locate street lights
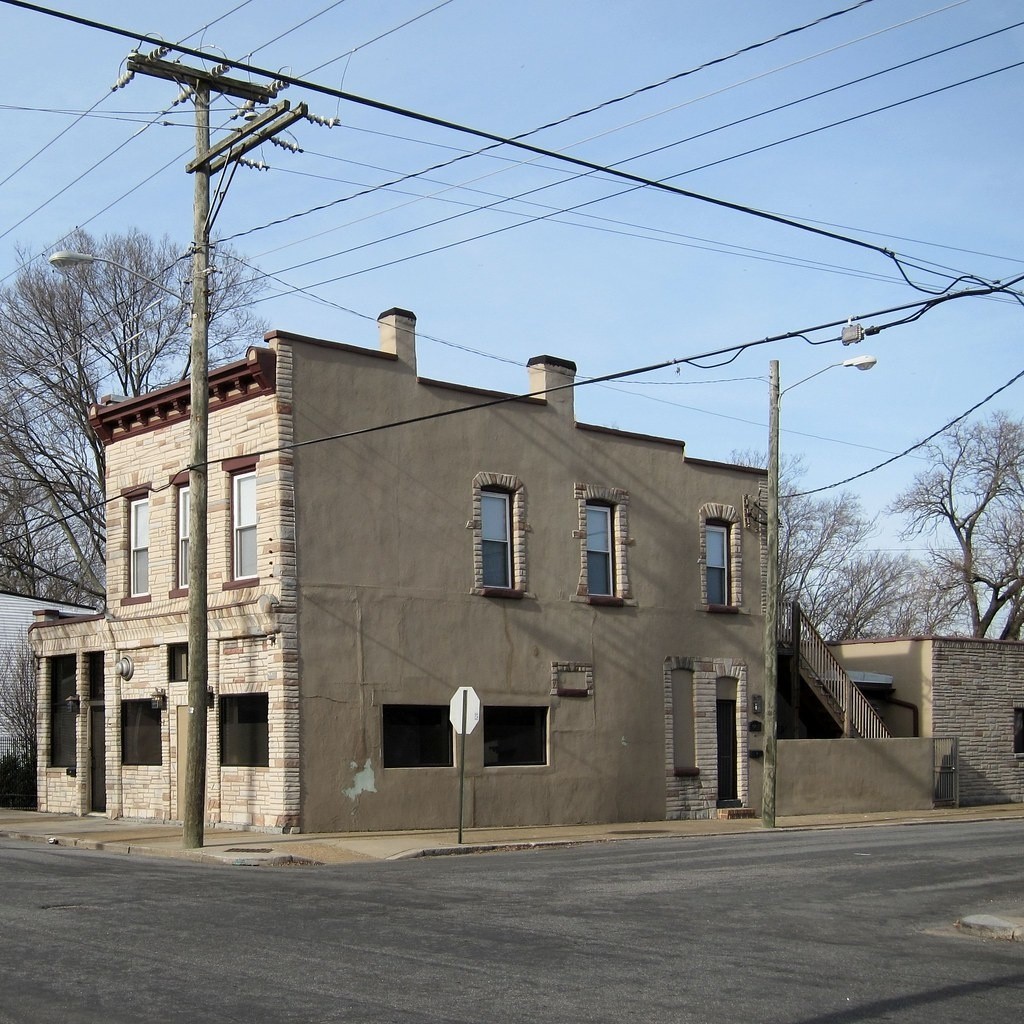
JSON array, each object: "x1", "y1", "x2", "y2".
[
  {"x1": 762, "y1": 355, "x2": 878, "y2": 829},
  {"x1": 45, "y1": 248, "x2": 212, "y2": 852}
]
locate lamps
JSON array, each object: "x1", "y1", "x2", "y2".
[
  {"x1": 66, "y1": 695, "x2": 79, "y2": 713},
  {"x1": 151, "y1": 689, "x2": 165, "y2": 708},
  {"x1": 205, "y1": 686, "x2": 213, "y2": 706}
]
